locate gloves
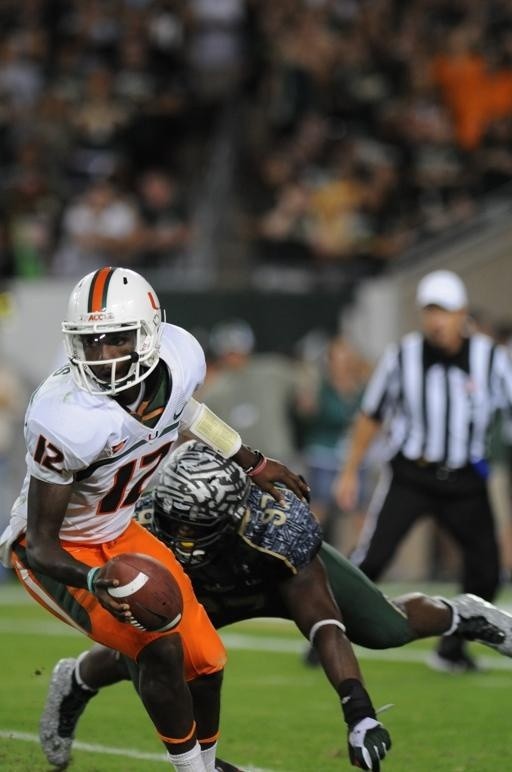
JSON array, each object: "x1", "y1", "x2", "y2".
[{"x1": 341, "y1": 696, "x2": 391, "y2": 771}]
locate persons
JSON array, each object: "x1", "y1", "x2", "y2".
[
  {"x1": 303, "y1": 266, "x2": 511, "y2": 673},
  {"x1": 0, "y1": 2, "x2": 511, "y2": 551},
  {"x1": 1, "y1": 265, "x2": 312, "y2": 772},
  {"x1": 36, "y1": 439, "x2": 512, "y2": 772}
]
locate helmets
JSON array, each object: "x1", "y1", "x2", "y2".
[
  {"x1": 152, "y1": 439, "x2": 252, "y2": 572},
  {"x1": 62, "y1": 266, "x2": 162, "y2": 397}
]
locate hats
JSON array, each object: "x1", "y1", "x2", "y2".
[{"x1": 418, "y1": 273, "x2": 467, "y2": 309}]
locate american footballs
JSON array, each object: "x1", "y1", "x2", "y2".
[{"x1": 106, "y1": 552, "x2": 183, "y2": 633}]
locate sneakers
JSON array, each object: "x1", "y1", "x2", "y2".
[
  {"x1": 444, "y1": 595, "x2": 512, "y2": 658},
  {"x1": 428, "y1": 649, "x2": 488, "y2": 675},
  {"x1": 38, "y1": 650, "x2": 99, "y2": 766}
]
